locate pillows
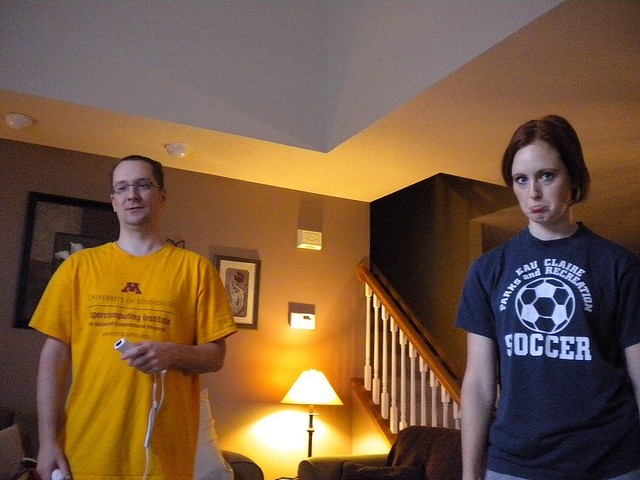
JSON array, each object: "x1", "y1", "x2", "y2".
[
  {"x1": 192, "y1": 389, "x2": 234, "y2": 480},
  {"x1": 342, "y1": 462, "x2": 411, "y2": 480},
  {"x1": 0, "y1": 425, "x2": 25, "y2": 480}
]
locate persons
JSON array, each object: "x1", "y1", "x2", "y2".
[
  {"x1": 455, "y1": 113, "x2": 635, "y2": 478},
  {"x1": 25, "y1": 153, "x2": 238, "y2": 475}
]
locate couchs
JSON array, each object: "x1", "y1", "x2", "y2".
[
  {"x1": 298, "y1": 424, "x2": 465, "y2": 480},
  {"x1": 1, "y1": 410, "x2": 265, "y2": 480}
]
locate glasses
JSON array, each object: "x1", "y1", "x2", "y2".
[{"x1": 110, "y1": 180, "x2": 161, "y2": 193}]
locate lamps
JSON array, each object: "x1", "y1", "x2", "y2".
[
  {"x1": 5, "y1": 113, "x2": 35, "y2": 131},
  {"x1": 281, "y1": 371, "x2": 344, "y2": 457},
  {"x1": 165, "y1": 142, "x2": 190, "y2": 158}
]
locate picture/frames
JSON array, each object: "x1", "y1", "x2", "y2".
[
  {"x1": 14, "y1": 194, "x2": 122, "y2": 330},
  {"x1": 213, "y1": 254, "x2": 260, "y2": 330}
]
